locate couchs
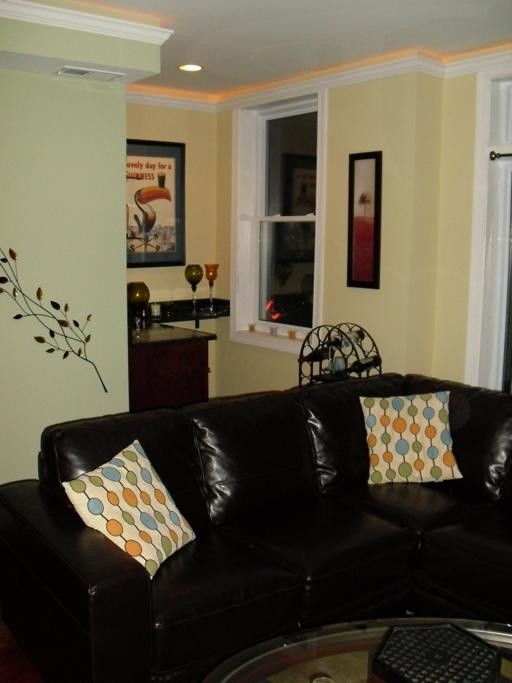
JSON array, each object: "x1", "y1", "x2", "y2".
[{"x1": 1, "y1": 372, "x2": 512, "y2": 682}]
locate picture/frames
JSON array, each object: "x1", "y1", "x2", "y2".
[
  {"x1": 347, "y1": 150, "x2": 381, "y2": 289},
  {"x1": 126, "y1": 138, "x2": 186, "y2": 267}
]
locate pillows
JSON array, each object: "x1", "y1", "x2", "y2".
[
  {"x1": 60, "y1": 438, "x2": 196, "y2": 579},
  {"x1": 359, "y1": 391, "x2": 463, "y2": 486}
]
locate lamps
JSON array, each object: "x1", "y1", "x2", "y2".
[
  {"x1": 204, "y1": 263, "x2": 219, "y2": 312},
  {"x1": 127, "y1": 282, "x2": 150, "y2": 341},
  {"x1": 185, "y1": 263, "x2": 203, "y2": 313}
]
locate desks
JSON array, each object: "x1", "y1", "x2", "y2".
[{"x1": 128, "y1": 322, "x2": 216, "y2": 413}]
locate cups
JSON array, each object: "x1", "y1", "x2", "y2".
[{"x1": 151, "y1": 301, "x2": 161, "y2": 317}]
